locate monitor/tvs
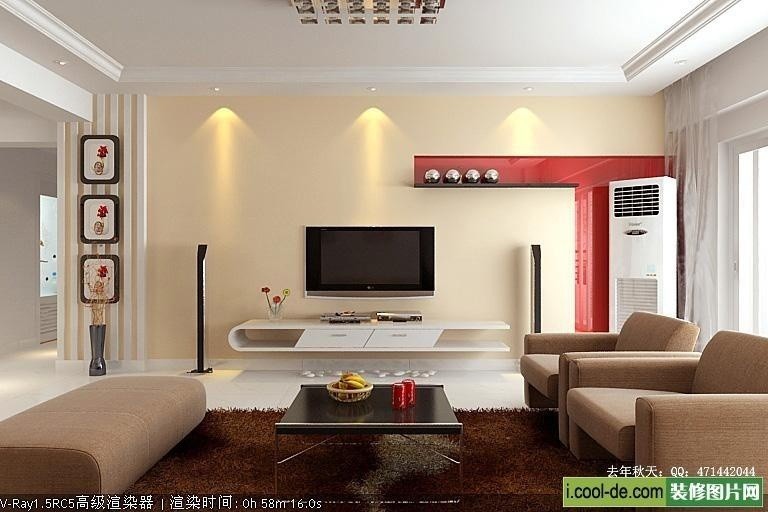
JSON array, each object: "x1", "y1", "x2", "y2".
[{"x1": 305, "y1": 226, "x2": 435, "y2": 300}]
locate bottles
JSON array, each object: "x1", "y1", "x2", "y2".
[{"x1": 392, "y1": 379, "x2": 416, "y2": 408}]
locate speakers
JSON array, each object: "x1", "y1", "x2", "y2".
[
  {"x1": 189, "y1": 245, "x2": 212, "y2": 373},
  {"x1": 530, "y1": 245, "x2": 541, "y2": 334}
]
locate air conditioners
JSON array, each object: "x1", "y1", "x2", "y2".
[{"x1": 609, "y1": 176, "x2": 678, "y2": 333}]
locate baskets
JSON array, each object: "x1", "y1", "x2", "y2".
[{"x1": 327, "y1": 379, "x2": 373, "y2": 402}]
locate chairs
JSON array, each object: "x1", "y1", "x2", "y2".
[
  {"x1": 566, "y1": 329, "x2": 768, "y2": 495},
  {"x1": 521, "y1": 311, "x2": 701, "y2": 448}
]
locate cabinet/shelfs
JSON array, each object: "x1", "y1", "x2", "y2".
[{"x1": 227, "y1": 319, "x2": 511, "y2": 353}]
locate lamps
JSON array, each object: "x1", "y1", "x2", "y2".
[{"x1": 290, "y1": 0, "x2": 446, "y2": 26}]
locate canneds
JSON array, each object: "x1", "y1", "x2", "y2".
[
  {"x1": 392, "y1": 383, "x2": 405, "y2": 410},
  {"x1": 402, "y1": 380, "x2": 416, "y2": 407}
]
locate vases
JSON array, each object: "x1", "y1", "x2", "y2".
[{"x1": 88, "y1": 324, "x2": 107, "y2": 376}]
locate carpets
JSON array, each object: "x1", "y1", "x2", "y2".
[{"x1": 112, "y1": 406, "x2": 636, "y2": 512}]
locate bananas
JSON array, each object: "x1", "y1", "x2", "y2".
[{"x1": 340, "y1": 373, "x2": 366, "y2": 389}]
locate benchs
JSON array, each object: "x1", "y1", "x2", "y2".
[{"x1": 0, "y1": 375, "x2": 207, "y2": 494}]
